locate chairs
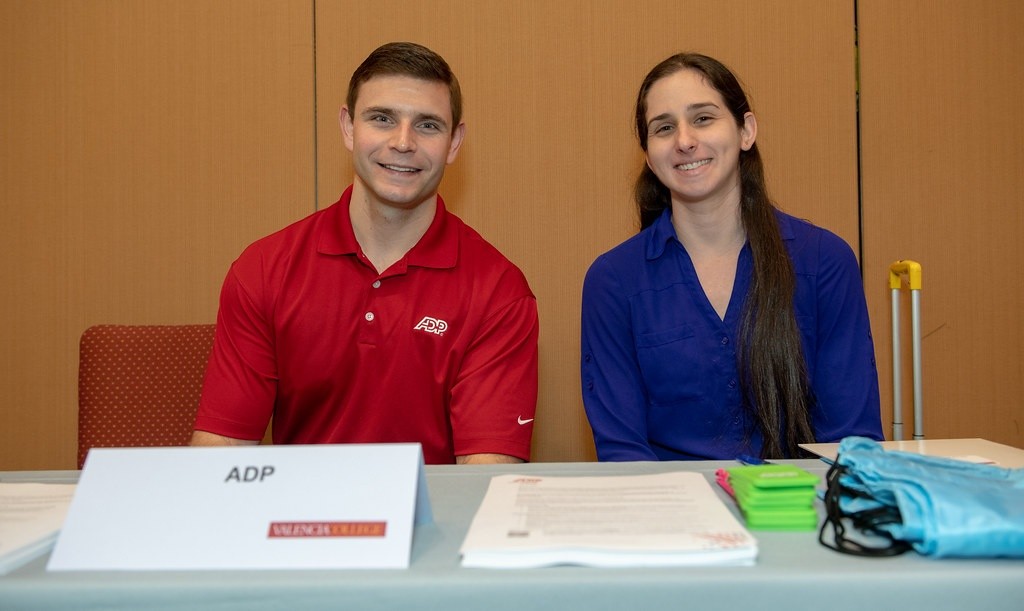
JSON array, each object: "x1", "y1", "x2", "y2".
[{"x1": 77, "y1": 324, "x2": 217, "y2": 470}]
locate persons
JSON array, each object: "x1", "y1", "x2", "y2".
[
  {"x1": 580, "y1": 52, "x2": 885, "y2": 462},
  {"x1": 193, "y1": 43, "x2": 540, "y2": 466}
]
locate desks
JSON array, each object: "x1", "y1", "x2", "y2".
[{"x1": 0, "y1": 437, "x2": 1024, "y2": 611}]
